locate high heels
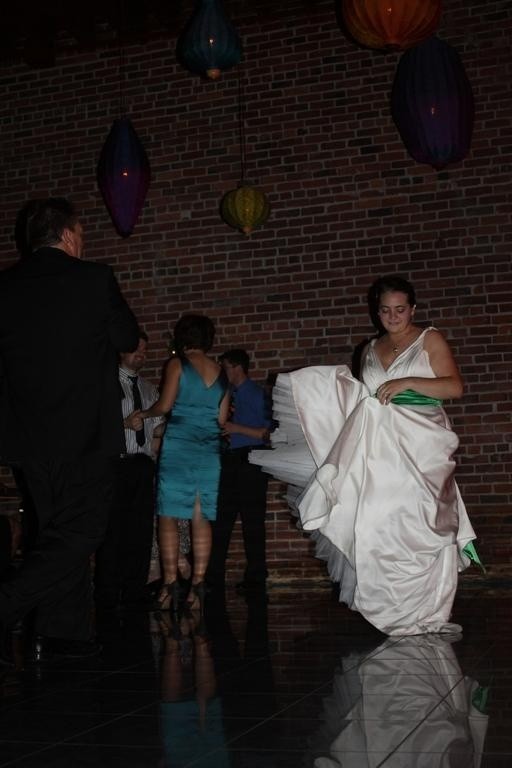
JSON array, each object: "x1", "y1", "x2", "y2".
[
  {"x1": 183, "y1": 579, "x2": 206, "y2": 614},
  {"x1": 152, "y1": 579, "x2": 181, "y2": 615}
]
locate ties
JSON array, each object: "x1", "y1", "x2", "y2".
[{"x1": 128, "y1": 375, "x2": 146, "y2": 447}]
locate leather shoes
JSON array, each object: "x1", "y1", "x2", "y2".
[{"x1": 235, "y1": 579, "x2": 265, "y2": 591}]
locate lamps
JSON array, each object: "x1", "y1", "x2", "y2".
[
  {"x1": 219, "y1": 181, "x2": 272, "y2": 237},
  {"x1": 340, "y1": 2, "x2": 444, "y2": 51},
  {"x1": 389, "y1": 33, "x2": 476, "y2": 169},
  {"x1": 173, "y1": 3, "x2": 242, "y2": 81},
  {"x1": 95, "y1": 116, "x2": 153, "y2": 241}
]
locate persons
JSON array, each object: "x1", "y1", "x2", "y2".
[
  {"x1": 250, "y1": 273, "x2": 489, "y2": 638},
  {"x1": 4, "y1": 197, "x2": 142, "y2": 678},
  {"x1": 111, "y1": 316, "x2": 277, "y2": 619},
  {"x1": 156, "y1": 609, "x2": 231, "y2": 767},
  {"x1": 307, "y1": 636, "x2": 494, "y2": 767},
  {"x1": 383, "y1": 334, "x2": 406, "y2": 354}
]
